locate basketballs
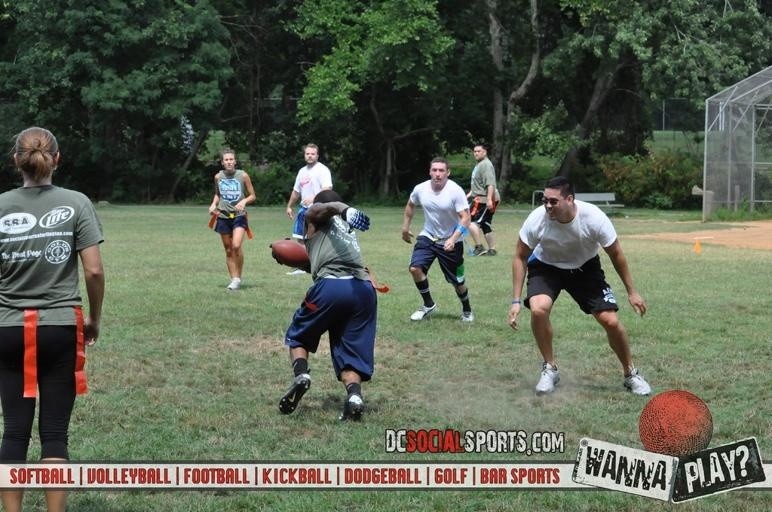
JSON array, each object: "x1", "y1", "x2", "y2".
[{"x1": 639, "y1": 389, "x2": 713, "y2": 457}]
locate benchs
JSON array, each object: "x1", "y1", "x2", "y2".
[{"x1": 533, "y1": 190, "x2": 625, "y2": 214}]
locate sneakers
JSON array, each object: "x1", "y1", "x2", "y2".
[
  {"x1": 473, "y1": 244, "x2": 487, "y2": 256},
  {"x1": 227, "y1": 279, "x2": 240, "y2": 289},
  {"x1": 279, "y1": 373, "x2": 311, "y2": 414},
  {"x1": 536, "y1": 362, "x2": 560, "y2": 394},
  {"x1": 488, "y1": 249, "x2": 496, "y2": 256},
  {"x1": 410, "y1": 302, "x2": 437, "y2": 321},
  {"x1": 286, "y1": 268, "x2": 306, "y2": 274},
  {"x1": 461, "y1": 312, "x2": 473, "y2": 321},
  {"x1": 624, "y1": 368, "x2": 651, "y2": 395},
  {"x1": 339, "y1": 392, "x2": 364, "y2": 421}
]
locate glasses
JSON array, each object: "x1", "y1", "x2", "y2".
[{"x1": 541, "y1": 196, "x2": 559, "y2": 205}]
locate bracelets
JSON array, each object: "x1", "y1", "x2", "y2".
[{"x1": 511, "y1": 298, "x2": 522, "y2": 304}]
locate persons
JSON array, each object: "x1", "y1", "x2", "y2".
[
  {"x1": 0, "y1": 126, "x2": 106, "y2": 512},
  {"x1": 209, "y1": 149, "x2": 256, "y2": 290},
  {"x1": 509, "y1": 178, "x2": 650, "y2": 397},
  {"x1": 402, "y1": 157, "x2": 473, "y2": 323},
  {"x1": 466, "y1": 145, "x2": 500, "y2": 256},
  {"x1": 286, "y1": 143, "x2": 333, "y2": 275},
  {"x1": 279, "y1": 189, "x2": 378, "y2": 422}
]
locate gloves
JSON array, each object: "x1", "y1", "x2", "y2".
[{"x1": 341, "y1": 207, "x2": 370, "y2": 233}]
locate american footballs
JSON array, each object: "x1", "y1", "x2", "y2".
[{"x1": 271, "y1": 240, "x2": 309, "y2": 267}]
